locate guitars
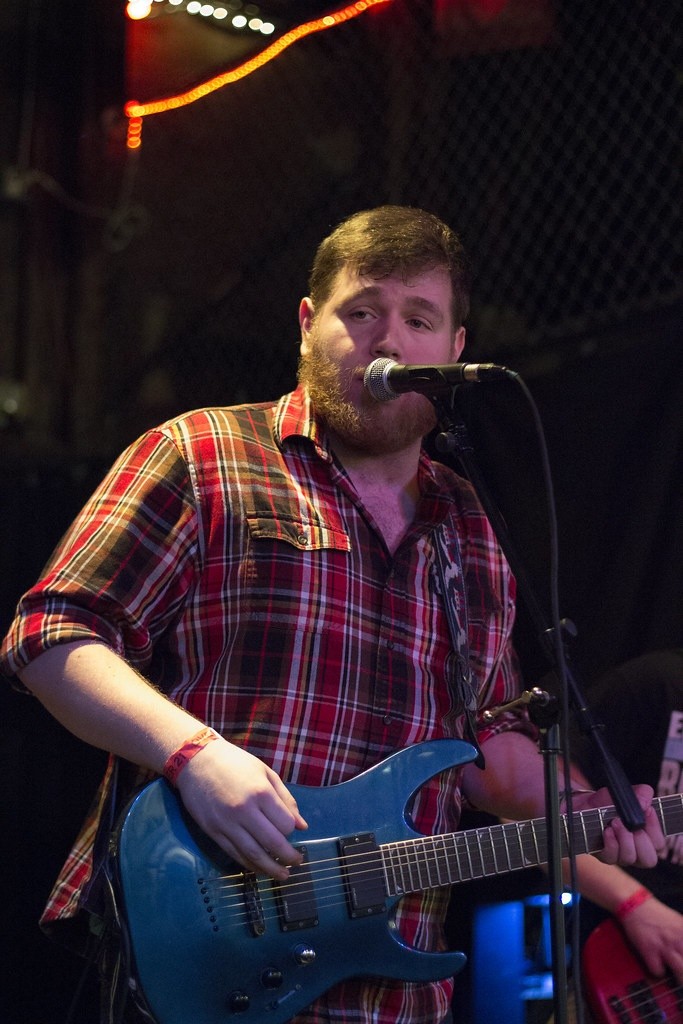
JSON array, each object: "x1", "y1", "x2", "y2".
[
  {"x1": 579, "y1": 899, "x2": 683, "y2": 1024},
  {"x1": 106, "y1": 737, "x2": 683, "y2": 1024}
]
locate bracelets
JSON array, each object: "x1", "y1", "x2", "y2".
[
  {"x1": 613, "y1": 886, "x2": 653, "y2": 919},
  {"x1": 163, "y1": 728, "x2": 216, "y2": 784}
]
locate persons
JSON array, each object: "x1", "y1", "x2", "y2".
[{"x1": 1, "y1": 202, "x2": 682, "y2": 1023}]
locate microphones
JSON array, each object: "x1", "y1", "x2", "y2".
[{"x1": 363, "y1": 357, "x2": 508, "y2": 402}]
